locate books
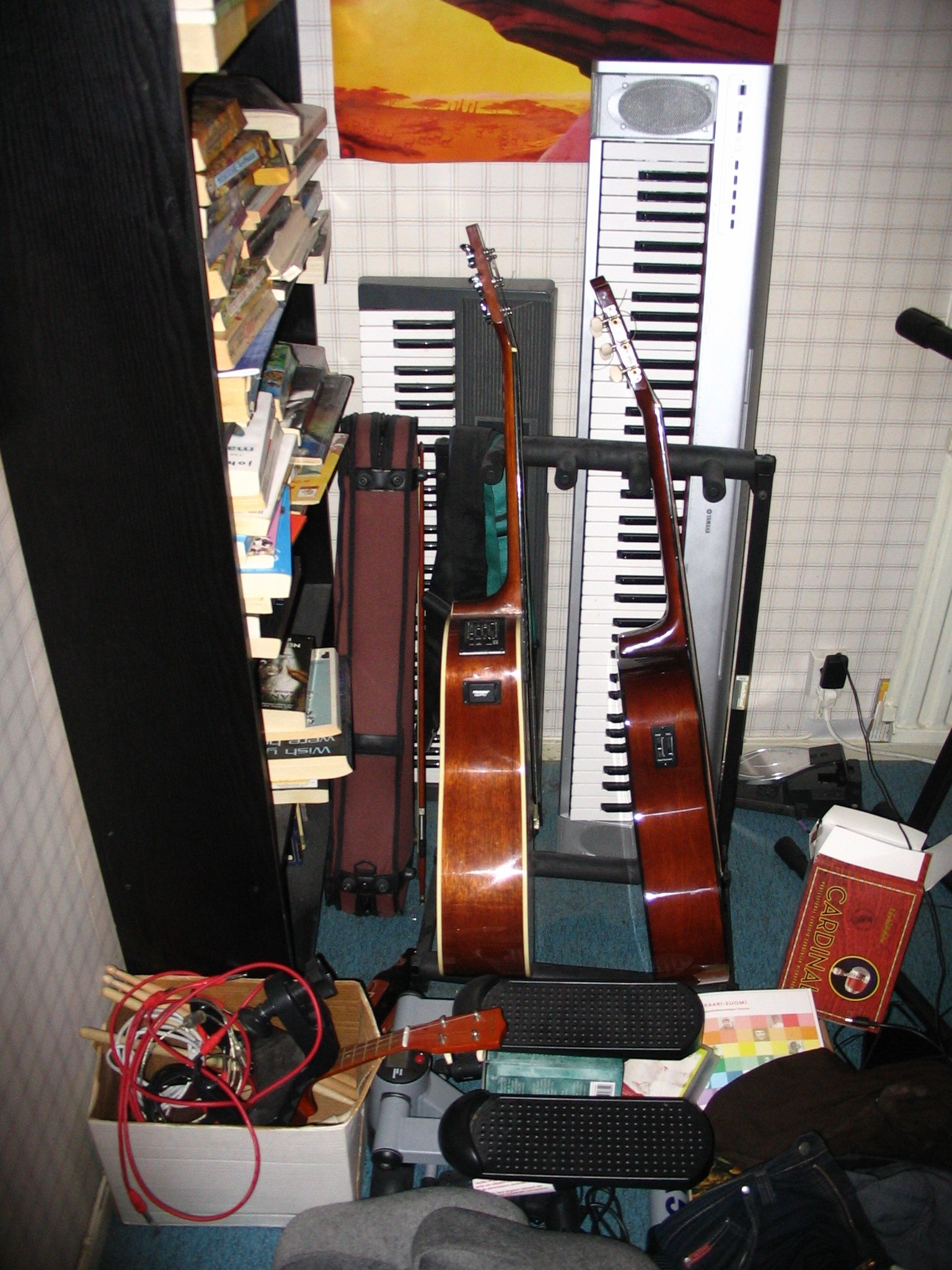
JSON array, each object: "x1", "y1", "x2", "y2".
[{"x1": 175, "y1": 0, "x2": 356, "y2": 806}]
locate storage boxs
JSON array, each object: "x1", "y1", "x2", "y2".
[
  {"x1": 85, "y1": 975, "x2": 385, "y2": 1228},
  {"x1": 779, "y1": 806, "x2": 951, "y2": 1034}
]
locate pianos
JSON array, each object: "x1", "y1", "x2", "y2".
[
  {"x1": 357, "y1": 276, "x2": 554, "y2": 805},
  {"x1": 560, "y1": 58, "x2": 773, "y2": 826}
]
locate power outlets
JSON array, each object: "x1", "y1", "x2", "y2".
[{"x1": 807, "y1": 650, "x2": 848, "y2": 699}]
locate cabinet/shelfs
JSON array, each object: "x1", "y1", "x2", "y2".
[{"x1": 1, "y1": 0, "x2": 332, "y2": 975}]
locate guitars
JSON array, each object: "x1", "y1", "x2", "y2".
[
  {"x1": 292, "y1": 1006, "x2": 507, "y2": 1124},
  {"x1": 434, "y1": 224, "x2": 539, "y2": 980},
  {"x1": 588, "y1": 276, "x2": 733, "y2": 985}
]
